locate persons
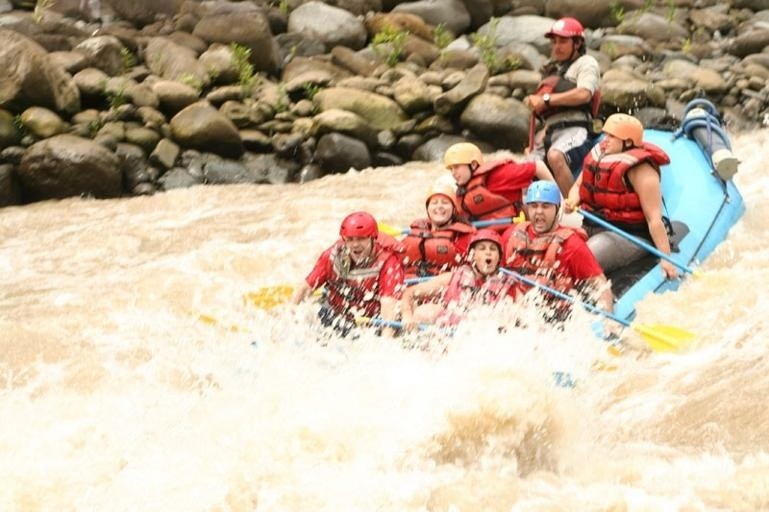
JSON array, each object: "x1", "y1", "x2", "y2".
[
  {"x1": 400, "y1": 185, "x2": 477, "y2": 307},
  {"x1": 503, "y1": 179, "x2": 622, "y2": 337},
  {"x1": 397, "y1": 230, "x2": 524, "y2": 351},
  {"x1": 523, "y1": 16, "x2": 601, "y2": 198},
  {"x1": 291, "y1": 211, "x2": 403, "y2": 339},
  {"x1": 443, "y1": 142, "x2": 564, "y2": 236},
  {"x1": 561, "y1": 114, "x2": 678, "y2": 282}
]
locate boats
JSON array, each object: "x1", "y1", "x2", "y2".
[{"x1": 529, "y1": 121, "x2": 754, "y2": 388}]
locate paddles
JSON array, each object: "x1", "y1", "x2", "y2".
[
  {"x1": 250, "y1": 282, "x2": 334, "y2": 313},
  {"x1": 575, "y1": 208, "x2": 725, "y2": 291},
  {"x1": 375, "y1": 208, "x2": 528, "y2": 242},
  {"x1": 492, "y1": 264, "x2": 693, "y2": 355}
]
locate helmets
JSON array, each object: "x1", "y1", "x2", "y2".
[
  {"x1": 443, "y1": 142, "x2": 483, "y2": 169},
  {"x1": 339, "y1": 212, "x2": 378, "y2": 239},
  {"x1": 544, "y1": 17, "x2": 585, "y2": 38},
  {"x1": 603, "y1": 113, "x2": 643, "y2": 147},
  {"x1": 426, "y1": 183, "x2": 458, "y2": 216},
  {"x1": 526, "y1": 180, "x2": 561, "y2": 210},
  {"x1": 467, "y1": 228, "x2": 502, "y2": 260}
]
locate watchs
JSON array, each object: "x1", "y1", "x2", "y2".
[{"x1": 543, "y1": 92, "x2": 551, "y2": 109}]
